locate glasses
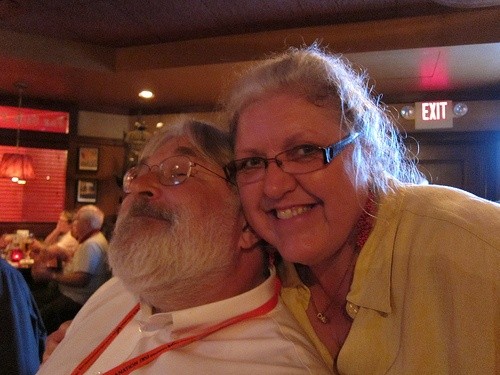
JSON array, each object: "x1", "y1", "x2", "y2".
[
  {"x1": 221, "y1": 132, "x2": 363, "y2": 185},
  {"x1": 119, "y1": 156, "x2": 237, "y2": 193}
]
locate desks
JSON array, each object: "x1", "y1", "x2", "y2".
[{"x1": 0, "y1": 236, "x2": 58, "y2": 301}]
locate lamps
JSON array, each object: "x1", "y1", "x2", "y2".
[
  {"x1": 125, "y1": 100, "x2": 153, "y2": 154},
  {"x1": 0, "y1": 82, "x2": 38, "y2": 181},
  {"x1": 400, "y1": 99, "x2": 468, "y2": 130}
]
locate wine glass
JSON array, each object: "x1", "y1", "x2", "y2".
[{"x1": 24, "y1": 237, "x2": 32, "y2": 259}]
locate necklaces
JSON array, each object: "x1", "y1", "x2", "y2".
[{"x1": 308, "y1": 243, "x2": 358, "y2": 324}]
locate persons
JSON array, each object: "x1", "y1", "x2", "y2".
[
  {"x1": 223, "y1": 44, "x2": 500, "y2": 375},
  {"x1": 36, "y1": 118, "x2": 332, "y2": 375},
  {"x1": 0, "y1": 205, "x2": 113, "y2": 375}
]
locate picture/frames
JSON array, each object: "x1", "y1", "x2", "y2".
[
  {"x1": 74, "y1": 177, "x2": 99, "y2": 205},
  {"x1": 78, "y1": 145, "x2": 101, "y2": 174}
]
